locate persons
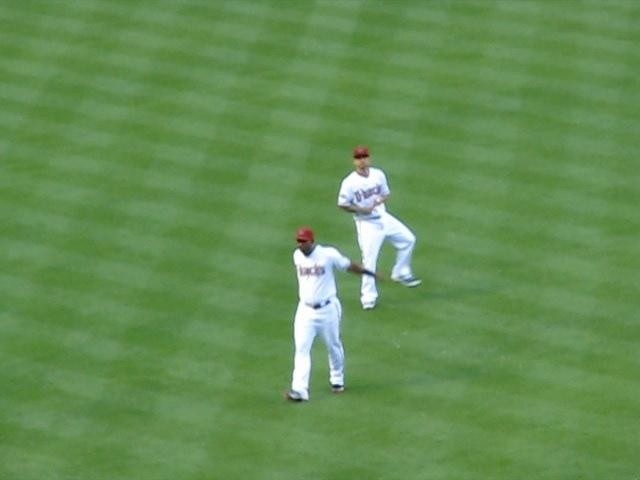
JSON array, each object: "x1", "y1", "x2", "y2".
[
  {"x1": 284, "y1": 228, "x2": 383, "y2": 401},
  {"x1": 337, "y1": 146, "x2": 424, "y2": 310}
]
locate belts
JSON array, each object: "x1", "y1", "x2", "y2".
[{"x1": 307, "y1": 301, "x2": 330, "y2": 309}]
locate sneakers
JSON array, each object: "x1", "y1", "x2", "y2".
[
  {"x1": 362, "y1": 302, "x2": 375, "y2": 309},
  {"x1": 285, "y1": 390, "x2": 301, "y2": 400},
  {"x1": 332, "y1": 384, "x2": 344, "y2": 392},
  {"x1": 391, "y1": 275, "x2": 422, "y2": 286}
]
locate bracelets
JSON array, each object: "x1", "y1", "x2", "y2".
[
  {"x1": 362, "y1": 270, "x2": 376, "y2": 278},
  {"x1": 375, "y1": 198, "x2": 381, "y2": 206}
]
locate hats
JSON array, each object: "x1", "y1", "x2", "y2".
[
  {"x1": 297, "y1": 229, "x2": 313, "y2": 241},
  {"x1": 353, "y1": 148, "x2": 369, "y2": 157}
]
